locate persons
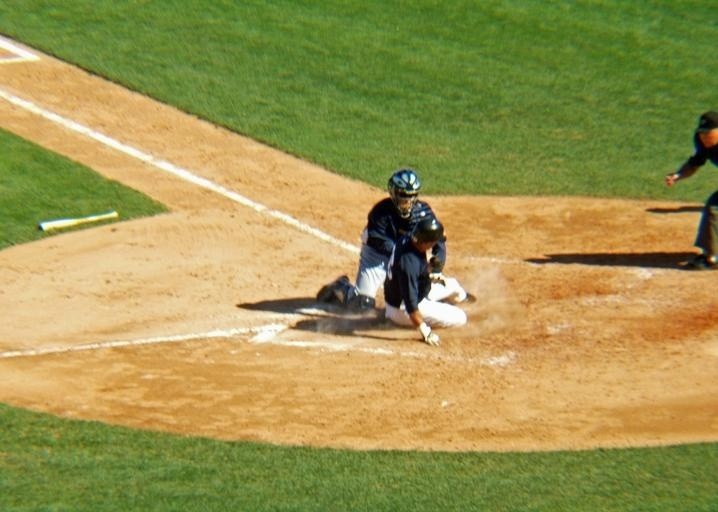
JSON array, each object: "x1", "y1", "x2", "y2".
[
  {"x1": 383, "y1": 216, "x2": 468, "y2": 346},
  {"x1": 664, "y1": 111, "x2": 718, "y2": 271},
  {"x1": 316, "y1": 168, "x2": 446, "y2": 315}
]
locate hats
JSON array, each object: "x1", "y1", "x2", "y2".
[{"x1": 695, "y1": 111, "x2": 718, "y2": 134}]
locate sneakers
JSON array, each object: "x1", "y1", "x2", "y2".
[
  {"x1": 316, "y1": 274, "x2": 350, "y2": 304},
  {"x1": 677, "y1": 253, "x2": 717, "y2": 269}
]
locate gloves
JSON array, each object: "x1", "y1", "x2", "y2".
[{"x1": 419, "y1": 323, "x2": 441, "y2": 347}]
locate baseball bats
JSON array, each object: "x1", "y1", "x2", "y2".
[{"x1": 39, "y1": 210, "x2": 119, "y2": 232}]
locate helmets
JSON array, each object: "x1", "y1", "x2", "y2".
[
  {"x1": 414, "y1": 218, "x2": 446, "y2": 244},
  {"x1": 387, "y1": 167, "x2": 421, "y2": 206}
]
